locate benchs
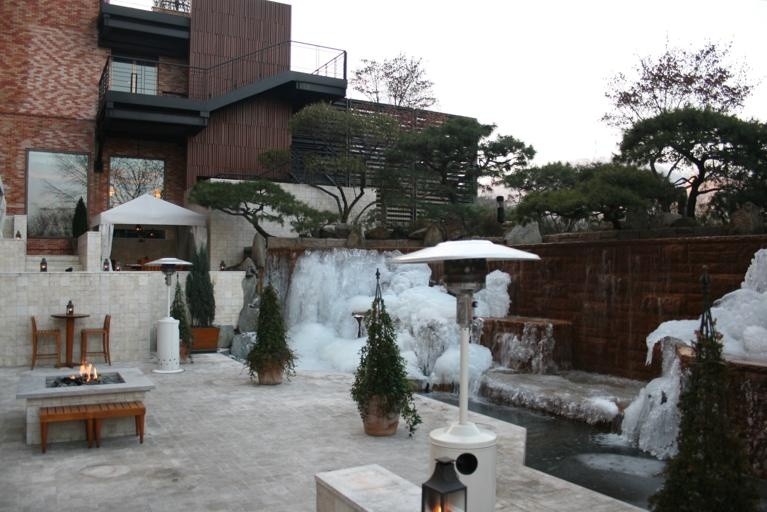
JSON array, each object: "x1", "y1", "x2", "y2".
[{"x1": 39, "y1": 400, "x2": 147, "y2": 454}]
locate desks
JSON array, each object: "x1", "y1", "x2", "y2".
[{"x1": 52, "y1": 313, "x2": 90, "y2": 369}]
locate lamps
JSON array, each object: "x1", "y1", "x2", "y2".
[
  {"x1": 420, "y1": 449, "x2": 479, "y2": 512},
  {"x1": 66, "y1": 300, "x2": 74, "y2": 315},
  {"x1": 40, "y1": 258, "x2": 48, "y2": 272}
]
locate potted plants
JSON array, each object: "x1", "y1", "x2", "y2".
[
  {"x1": 172, "y1": 244, "x2": 223, "y2": 366},
  {"x1": 353, "y1": 297, "x2": 423, "y2": 437},
  {"x1": 246, "y1": 281, "x2": 299, "y2": 385}
]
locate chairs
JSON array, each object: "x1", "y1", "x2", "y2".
[
  {"x1": 30, "y1": 316, "x2": 61, "y2": 370},
  {"x1": 81, "y1": 315, "x2": 113, "y2": 367}
]
